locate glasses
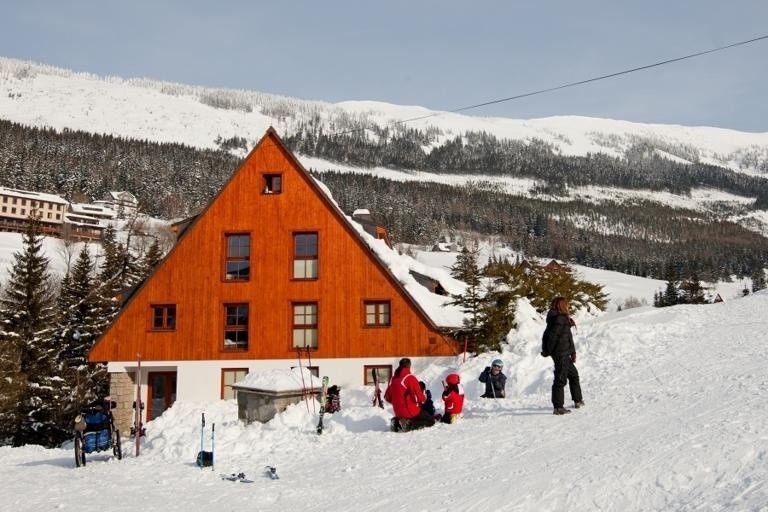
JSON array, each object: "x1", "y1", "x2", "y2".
[{"x1": 493, "y1": 364, "x2": 501, "y2": 368}]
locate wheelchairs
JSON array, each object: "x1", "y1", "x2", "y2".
[{"x1": 72, "y1": 394, "x2": 122, "y2": 468}]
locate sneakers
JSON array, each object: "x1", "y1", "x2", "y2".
[
  {"x1": 391, "y1": 418, "x2": 397, "y2": 432},
  {"x1": 575, "y1": 401, "x2": 584, "y2": 408},
  {"x1": 553, "y1": 407, "x2": 570, "y2": 414},
  {"x1": 398, "y1": 417, "x2": 409, "y2": 432}
]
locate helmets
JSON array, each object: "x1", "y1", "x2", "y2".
[{"x1": 492, "y1": 360, "x2": 504, "y2": 367}]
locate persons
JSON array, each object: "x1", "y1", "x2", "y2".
[
  {"x1": 383, "y1": 358, "x2": 435, "y2": 432},
  {"x1": 479, "y1": 359, "x2": 507, "y2": 398},
  {"x1": 546, "y1": 296, "x2": 586, "y2": 414},
  {"x1": 418, "y1": 380, "x2": 433, "y2": 417},
  {"x1": 441, "y1": 374, "x2": 465, "y2": 424}
]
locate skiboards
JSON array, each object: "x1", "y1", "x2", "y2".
[
  {"x1": 221, "y1": 464, "x2": 280, "y2": 484},
  {"x1": 316, "y1": 377, "x2": 329, "y2": 434},
  {"x1": 371, "y1": 367, "x2": 385, "y2": 408}
]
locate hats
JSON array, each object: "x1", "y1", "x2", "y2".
[{"x1": 446, "y1": 374, "x2": 460, "y2": 385}]
